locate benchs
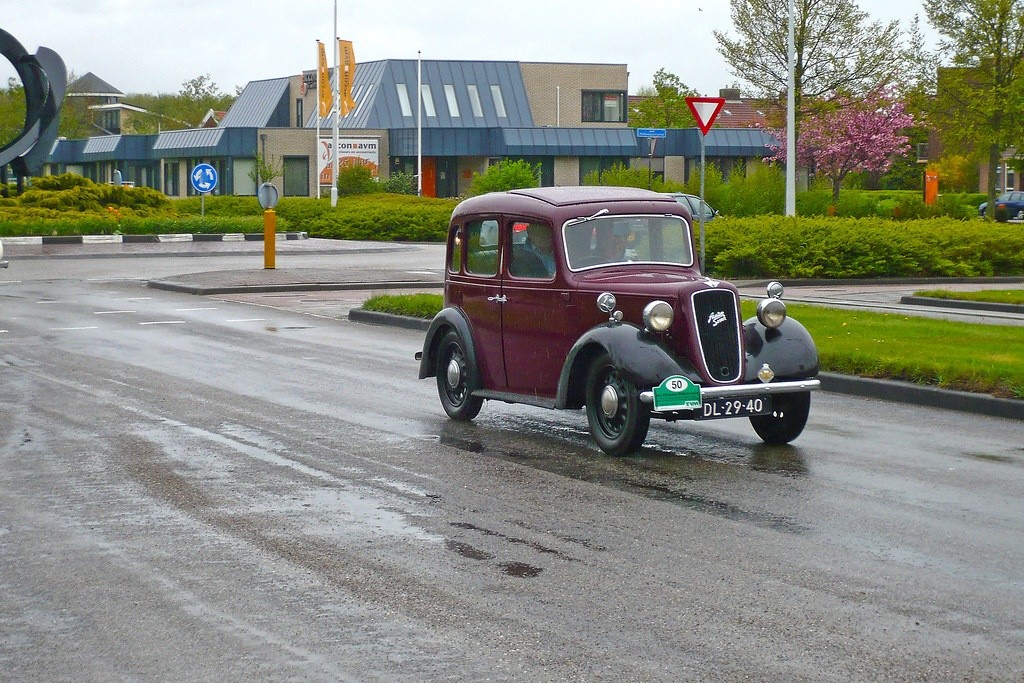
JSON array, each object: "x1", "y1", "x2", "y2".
[{"x1": 469, "y1": 250, "x2": 548, "y2": 279}]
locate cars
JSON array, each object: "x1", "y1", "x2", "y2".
[
  {"x1": 413, "y1": 186, "x2": 821, "y2": 457},
  {"x1": 482, "y1": 219, "x2": 528, "y2": 245},
  {"x1": 978, "y1": 190, "x2": 1024, "y2": 220}
]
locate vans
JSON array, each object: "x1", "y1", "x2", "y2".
[{"x1": 629, "y1": 192, "x2": 721, "y2": 233}]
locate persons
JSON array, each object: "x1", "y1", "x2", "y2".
[{"x1": 518, "y1": 222, "x2": 556, "y2": 277}]
